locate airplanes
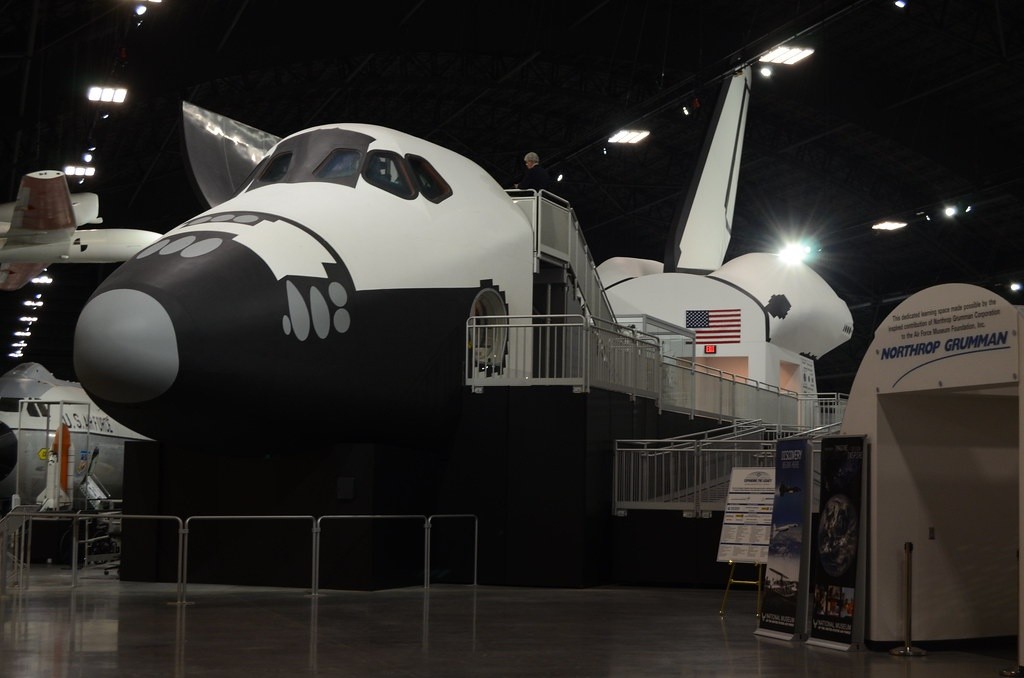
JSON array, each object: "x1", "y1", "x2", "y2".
[
  {"x1": 1, "y1": 44, "x2": 859, "y2": 590},
  {"x1": 0, "y1": 362, "x2": 157, "y2": 571}
]
